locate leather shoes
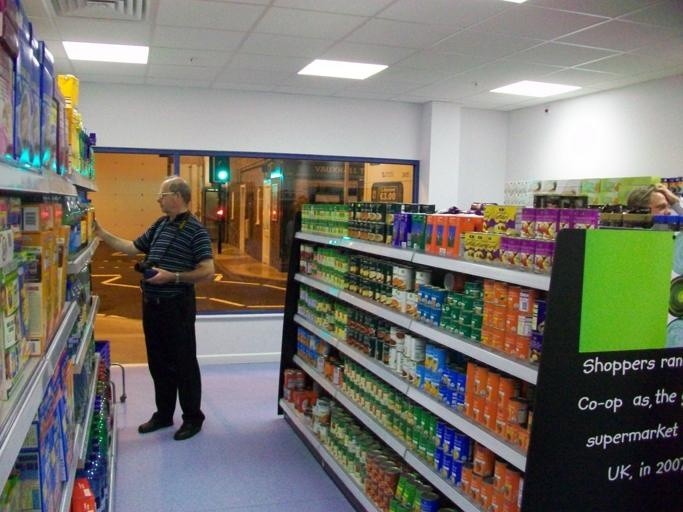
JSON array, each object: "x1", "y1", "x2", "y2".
[
  {"x1": 172, "y1": 424, "x2": 199, "y2": 440},
  {"x1": 137, "y1": 417, "x2": 171, "y2": 434}
]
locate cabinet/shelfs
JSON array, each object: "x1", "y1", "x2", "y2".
[
  {"x1": 0, "y1": 133, "x2": 114, "y2": 512},
  {"x1": 277, "y1": 209, "x2": 683, "y2": 511}
]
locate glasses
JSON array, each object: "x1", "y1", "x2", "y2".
[{"x1": 155, "y1": 189, "x2": 176, "y2": 203}]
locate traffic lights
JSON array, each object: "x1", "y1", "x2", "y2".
[{"x1": 209, "y1": 156, "x2": 231, "y2": 184}]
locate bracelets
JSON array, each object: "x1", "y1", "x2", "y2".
[{"x1": 175, "y1": 272, "x2": 180, "y2": 285}]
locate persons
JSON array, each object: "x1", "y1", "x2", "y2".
[
  {"x1": 626, "y1": 183, "x2": 682, "y2": 217},
  {"x1": 93, "y1": 176, "x2": 216, "y2": 440}
]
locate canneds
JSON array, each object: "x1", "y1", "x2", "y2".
[{"x1": 284, "y1": 196, "x2": 600, "y2": 512}]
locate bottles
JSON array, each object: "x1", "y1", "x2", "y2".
[{"x1": 75, "y1": 355, "x2": 113, "y2": 511}]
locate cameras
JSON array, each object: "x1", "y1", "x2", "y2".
[{"x1": 135, "y1": 260, "x2": 158, "y2": 274}]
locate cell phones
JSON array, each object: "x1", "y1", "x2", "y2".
[{"x1": 144, "y1": 269, "x2": 157, "y2": 279}]
